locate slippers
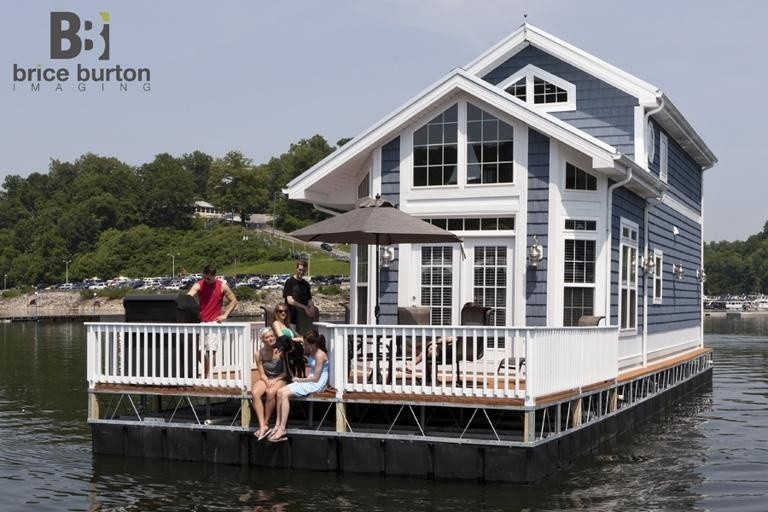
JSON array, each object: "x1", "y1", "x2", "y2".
[{"x1": 254, "y1": 427, "x2": 288, "y2": 443}]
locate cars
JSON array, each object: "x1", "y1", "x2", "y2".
[
  {"x1": 320, "y1": 243, "x2": 332, "y2": 251},
  {"x1": 703, "y1": 292, "x2": 768, "y2": 311},
  {"x1": 35, "y1": 272, "x2": 350, "y2": 292}
]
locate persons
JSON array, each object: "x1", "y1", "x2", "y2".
[
  {"x1": 282, "y1": 260, "x2": 316, "y2": 336},
  {"x1": 249, "y1": 326, "x2": 289, "y2": 442},
  {"x1": 187, "y1": 264, "x2": 239, "y2": 378},
  {"x1": 394, "y1": 334, "x2": 458, "y2": 384},
  {"x1": 271, "y1": 301, "x2": 306, "y2": 347},
  {"x1": 267, "y1": 329, "x2": 330, "y2": 442}
]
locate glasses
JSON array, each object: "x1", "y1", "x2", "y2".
[
  {"x1": 277, "y1": 310, "x2": 288, "y2": 313},
  {"x1": 203, "y1": 276, "x2": 214, "y2": 281}
]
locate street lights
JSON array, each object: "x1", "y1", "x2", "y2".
[
  {"x1": 301, "y1": 251, "x2": 318, "y2": 276},
  {"x1": 63, "y1": 260, "x2": 72, "y2": 284},
  {"x1": 4, "y1": 274, "x2": 8, "y2": 289},
  {"x1": 167, "y1": 252, "x2": 180, "y2": 278}
]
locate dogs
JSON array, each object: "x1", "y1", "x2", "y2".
[{"x1": 272, "y1": 336, "x2": 307, "y2": 378}]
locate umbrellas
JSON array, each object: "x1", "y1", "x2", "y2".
[{"x1": 286, "y1": 194, "x2": 464, "y2": 326}]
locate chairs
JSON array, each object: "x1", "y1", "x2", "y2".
[
  {"x1": 578, "y1": 316, "x2": 605, "y2": 328},
  {"x1": 497, "y1": 357, "x2": 526, "y2": 385},
  {"x1": 258, "y1": 302, "x2": 495, "y2": 386}
]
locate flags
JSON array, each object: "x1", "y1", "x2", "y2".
[{"x1": 222, "y1": 175, "x2": 233, "y2": 185}]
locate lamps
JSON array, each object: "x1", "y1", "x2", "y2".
[
  {"x1": 381, "y1": 245, "x2": 395, "y2": 267},
  {"x1": 673, "y1": 262, "x2": 685, "y2": 279},
  {"x1": 695, "y1": 268, "x2": 707, "y2": 282},
  {"x1": 645, "y1": 255, "x2": 655, "y2": 274},
  {"x1": 529, "y1": 234, "x2": 543, "y2": 267}
]
